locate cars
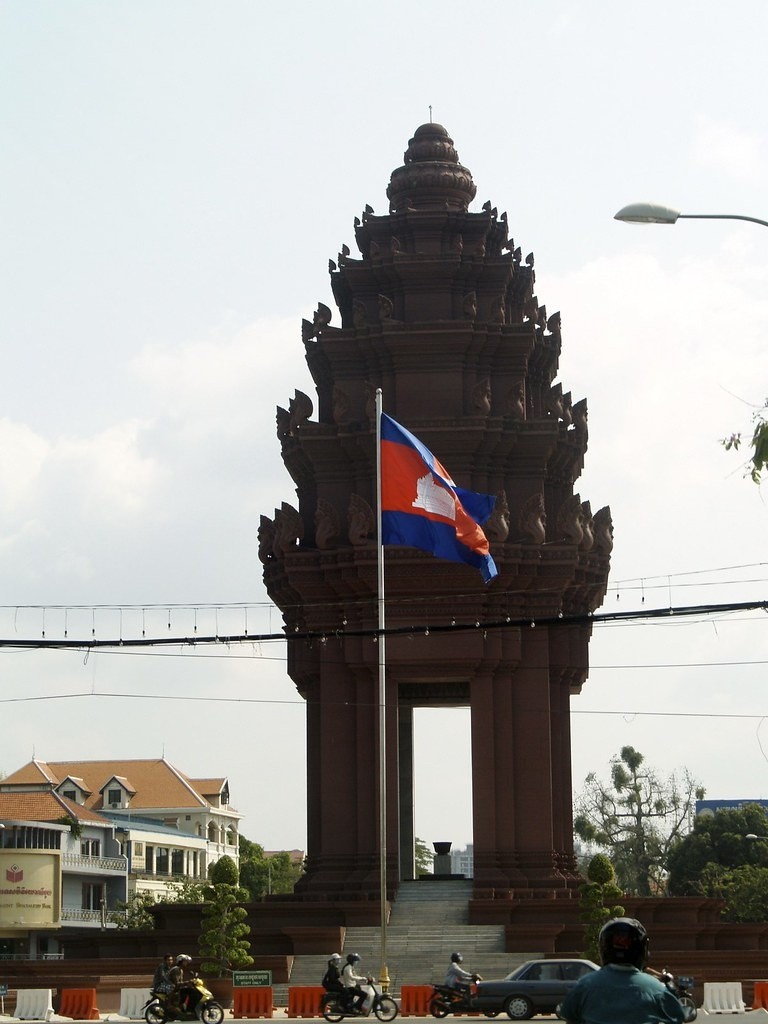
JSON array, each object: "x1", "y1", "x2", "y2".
[{"x1": 470, "y1": 958, "x2": 601, "y2": 1020}]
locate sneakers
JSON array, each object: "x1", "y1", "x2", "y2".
[{"x1": 351, "y1": 1007, "x2": 363, "y2": 1015}]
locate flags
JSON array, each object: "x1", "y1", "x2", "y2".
[{"x1": 380, "y1": 411, "x2": 500, "y2": 583}]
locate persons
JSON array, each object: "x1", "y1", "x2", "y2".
[
  {"x1": 153, "y1": 953, "x2": 192, "y2": 1015},
  {"x1": 556, "y1": 918, "x2": 696, "y2": 1024},
  {"x1": 446, "y1": 952, "x2": 476, "y2": 1007},
  {"x1": 322, "y1": 951, "x2": 376, "y2": 1013}
]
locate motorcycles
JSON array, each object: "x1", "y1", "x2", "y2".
[{"x1": 660, "y1": 970, "x2": 697, "y2": 1023}]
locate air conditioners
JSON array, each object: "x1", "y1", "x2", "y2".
[{"x1": 111, "y1": 802, "x2": 123, "y2": 809}]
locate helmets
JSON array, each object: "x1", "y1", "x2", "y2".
[
  {"x1": 331, "y1": 953, "x2": 342, "y2": 960},
  {"x1": 346, "y1": 953, "x2": 362, "y2": 963},
  {"x1": 175, "y1": 954, "x2": 192, "y2": 964},
  {"x1": 598, "y1": 917, "x2": 650, "y2": 971},
  {"x1": 451, "y1": 952, "x2": 463, "y2": 963}
]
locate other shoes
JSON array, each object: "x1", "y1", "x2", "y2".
[{"x1": 179, "y1": 1004, "x2": 187, "y2": 1014}]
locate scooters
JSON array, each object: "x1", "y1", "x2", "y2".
[
  {"x1": 426, "y1": 971, "x2": 499, "y2": 1018},
  {"x1": 318, "y1": 969, "x2": 398, "y2": 1023},
  {"x1": 140, "y1": 970, "x2": 224, "y2": 1024}
]
